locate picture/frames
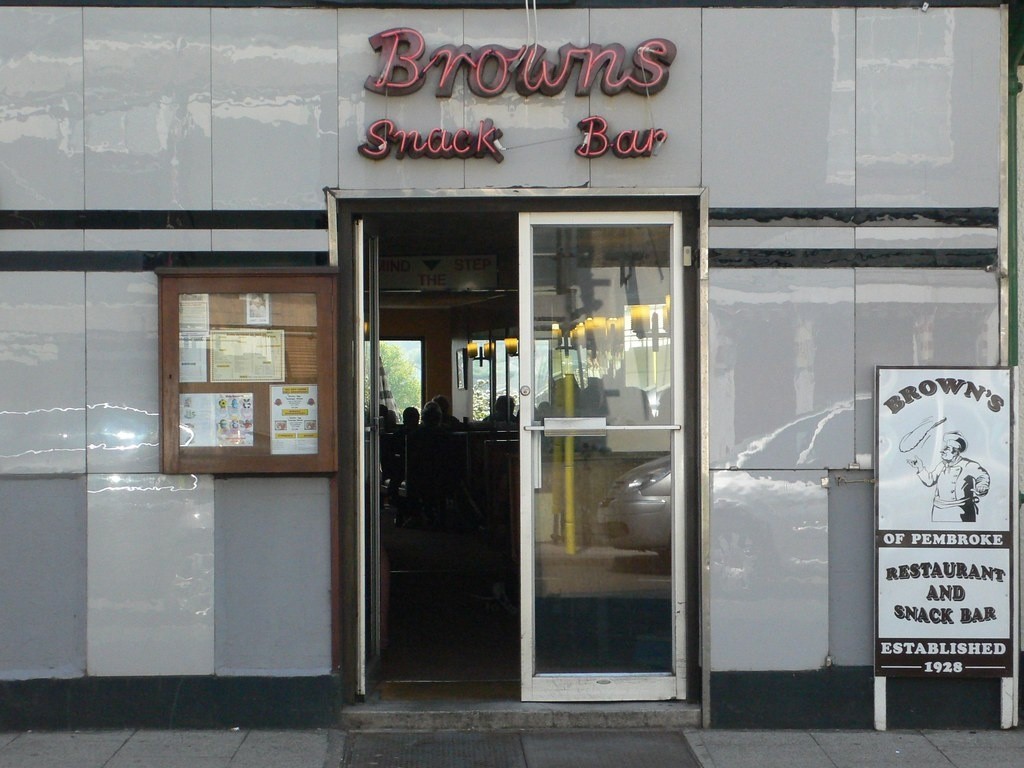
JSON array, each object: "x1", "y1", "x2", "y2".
[{"x1": 152, "y1": 263, "x2": 342, "y2": 475}]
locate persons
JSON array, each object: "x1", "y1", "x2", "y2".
[
  {"x1": 371, "y1": 393, "x2": 552, "y2": 526},
  {"x1": 250, "y1": 295, "x2": 266, "y2": 318}
]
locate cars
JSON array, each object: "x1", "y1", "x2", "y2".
[{"x1": 596, "y1": 390, "x2": 877, "y2": 585}]
